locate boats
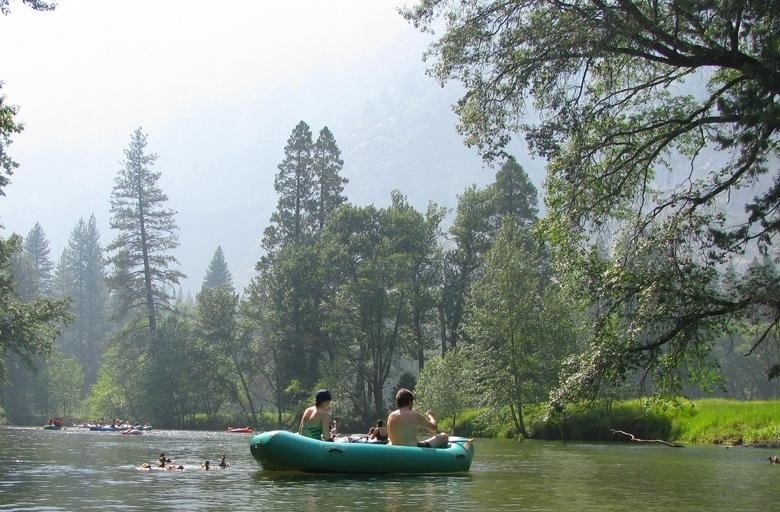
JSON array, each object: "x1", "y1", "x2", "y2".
[
  {"x1": 249, "y1": 430, "x2": 472, "y2": 476},
  {"x1": 42, "y1": 424, "x2": 62, "y2": 430},
  {"x1": 227, "y1": 426, "x2": 253, "y2": 433},
  {"x1": 73, "y1": 423, "x2": 152, "y2": 435}
]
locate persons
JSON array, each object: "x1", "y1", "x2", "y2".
[
  {"x1": 385, "y1": 386, "x2": 449, "y2": 449},
  {"x1": 47, "y1": 415, "x2": 152, "y2": 435},
  {"x1": 368, "y1": 419, "x2": 388, "y2": 440},
  {"x1": 298, "y1": 389, "x2": 335, "y2": 442}
]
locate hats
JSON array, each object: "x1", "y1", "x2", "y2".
[
  {"x1": 376, "y1": 420, "x2": 385, "y2": 424},
  {"x1": 315, "y1": 389, "x2": 332, "y2": 402}
]
